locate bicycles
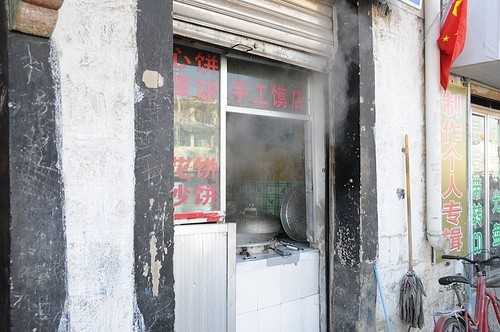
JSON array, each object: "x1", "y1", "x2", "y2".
[{"x1": 432, "y1": 249, "x2": 500, "y2": 332}]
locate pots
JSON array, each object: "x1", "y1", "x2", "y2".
[
  {"x1": 226, "y1": 207, "x2": 282, "y2": 237},
  {"x1": 236, "y1": 233, "x2": 277, "y2": 255}
]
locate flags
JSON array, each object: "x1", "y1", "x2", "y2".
[{"x1": 436, "y1": 0, "x2": 467, "y2": 91}]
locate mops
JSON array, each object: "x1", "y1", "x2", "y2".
[{"x1": 394, "y1": 134, "x2": 427, "y2": 330}]
